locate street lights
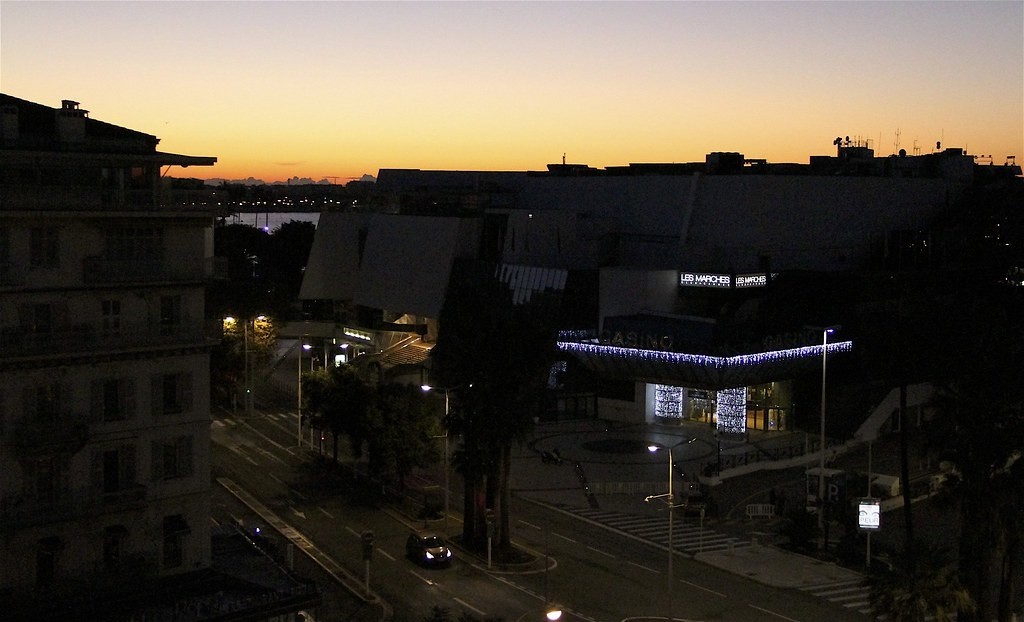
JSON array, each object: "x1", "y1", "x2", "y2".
[
  {"x1": 297, "y1": 327, "x2": 312, "y2": 446},
  {"x1": 818, "y1": 328, "x2": 838, "y2": 524},
  {"x1": 251, "y1": 310, "x2": 266, "y2": 409},
  {"x1": 226, "y1": 314, "x2": 247, "y2": 412},
  {"x1": 420, "y1": 384, "x2": 473, "y2": 531},
  {"x1": 647, "y1": 434, "x2": 700, "y2": 582},
  {"x1": 303, "y1": 345, "x2": 327, "y2": 373}
]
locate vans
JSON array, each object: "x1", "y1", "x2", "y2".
[{"x1": 405, "y1": 529, "x2": 450, "y2": 569}]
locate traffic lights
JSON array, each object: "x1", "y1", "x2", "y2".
[
  {"x1": 321, "y1": 437, "x2": 326, "y2": 441},
  {"x1": 231, "y1": 383, "x2": 238, "y2": 394},
  {"x1": 246, "y1": 388, "x2": 252, "y2": 394}
]
